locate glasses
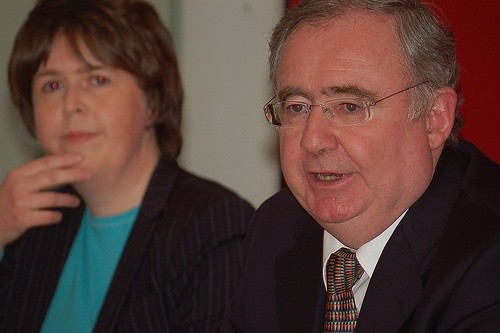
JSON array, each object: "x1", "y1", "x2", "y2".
[{"x1": 263, "y1": 80, "x2": 430, "y2": 125}]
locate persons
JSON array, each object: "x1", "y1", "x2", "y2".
[
  {"x1": 0, "y1": 0, "x2": 258, "y2": 333},
  {"x1": 220, "y1": 0, "x2": 500, "y2": 333}
]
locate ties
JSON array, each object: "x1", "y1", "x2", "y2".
[{"x1": 323, "y1": 248, "x2": 364, "y2": 333}]
